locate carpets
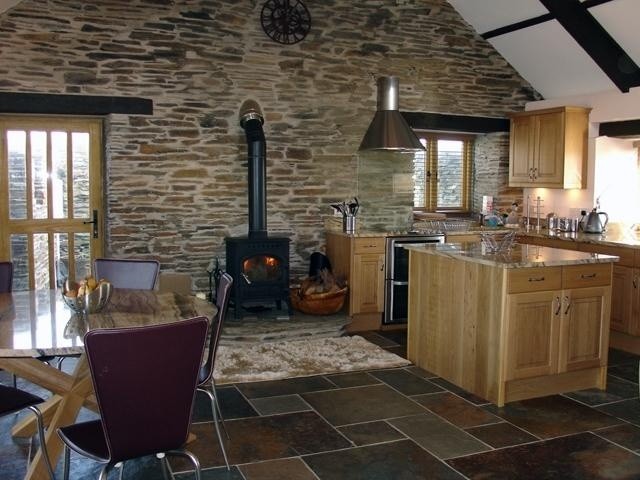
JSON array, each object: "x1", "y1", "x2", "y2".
[{"x1": 200, "y1": 312, "x2": 412, "y2": 387}]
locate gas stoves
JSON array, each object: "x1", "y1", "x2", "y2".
[{"x1": 377, "y1": 228, "x2": 443, "y2": 235}]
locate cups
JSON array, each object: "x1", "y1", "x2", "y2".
[{"x1": 550, "y1": 217, "x2": 578, "y2": 232}]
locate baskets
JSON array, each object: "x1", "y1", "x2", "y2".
[{"x1": 289, "y1": 278, "x2": 348, "y2": 316}]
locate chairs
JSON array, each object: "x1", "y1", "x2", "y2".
[
  {"x1": 0, "y1": 260, "x2": 56, "y2": 389},
  {"x1": 54, "y1": 316, "x2": 202, "y2": 479},
  {"x1": 192, "y1": 272, "x2": 233, "y2": 471},
  {"x1": 58, "y1": 259, "x2": 161, "y2": 374},
  {"x1": 1, "y1": 384, "x2": 58, "y2": 479}
]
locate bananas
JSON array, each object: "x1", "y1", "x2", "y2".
[{"x1": 78, "y1": 278, "x2": 92, "y2": 297}]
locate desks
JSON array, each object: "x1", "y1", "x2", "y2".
[{"x1": 0, "y1": 289, "x2": 218, "y2": 479}]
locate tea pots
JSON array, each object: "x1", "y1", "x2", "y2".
[{"x1": 579, "y1": 209, "x2": 608, "y2": 233}]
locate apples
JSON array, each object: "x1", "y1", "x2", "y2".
[{"x1": 88, "y1": 278, "x2": 97, "y2": 290}]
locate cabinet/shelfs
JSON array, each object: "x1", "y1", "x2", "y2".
[
  {"x1": 588, "y1": 243, "x2": 640, "y2": 355},
  {"x1": 499, "y1": 262, "x2": 613, "y2": 409},
  {"x1": 325, "y1": 233, "x2": 385, "y2": 316},
  {"x1": 505, "y1": 106, "x2": 593, "y2": 189}
]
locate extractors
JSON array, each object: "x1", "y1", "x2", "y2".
[{"x1": 358, "y1": 77, "x2": 427, "y2": 153}]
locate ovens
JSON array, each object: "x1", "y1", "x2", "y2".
[{"x1": 383, "y1": 235, "x2": 445, "y2": 326}]
